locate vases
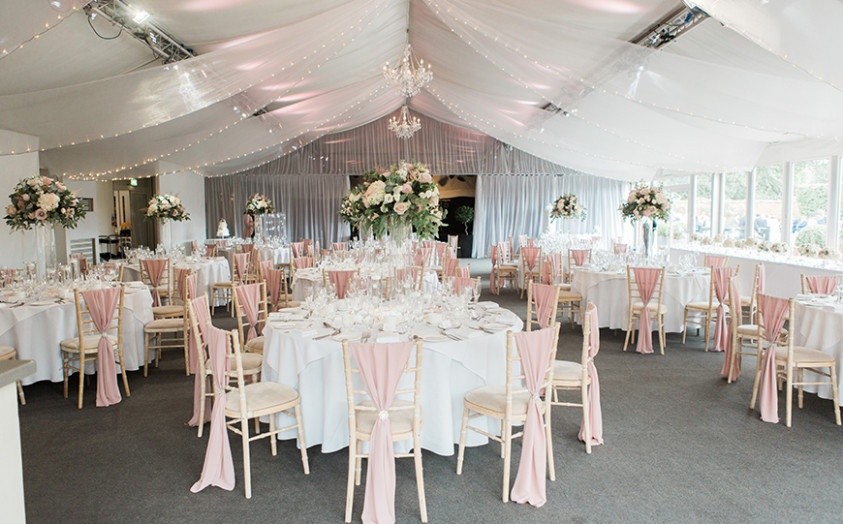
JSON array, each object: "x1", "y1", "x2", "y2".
[
  {"x1": 634, "y1": 215, "x2": 655, "y2": 268},
  {"x1": 562, "y1": 218, "x2": 574, "y2": 248},
  {"x1": 357, "y1": 213, "x2": 415, "y2": 275},
  {"x1": 160, "y1": 218, "x2": 173, "y2": 255},
  {"x1": 35, "y1": 223, "x2": 59, "y2": 286}
]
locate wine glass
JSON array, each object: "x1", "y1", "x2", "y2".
[{"x1": 0, "y1": 232, "x2": 708, "y2": 335}]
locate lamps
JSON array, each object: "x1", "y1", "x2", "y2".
[
  {"x1": 386, "y1": 96, "x2": 422, "y2": 140},
  {"x1": 82, "y1": 3, "x2": 98, "y2": 21},
  {"x1": 381, "y1": 27, "x2": 434, "y2": 99}
]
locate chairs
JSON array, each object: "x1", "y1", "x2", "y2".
[{"x1": 2, "y1": 229, "x2": 842, "y2": 522}]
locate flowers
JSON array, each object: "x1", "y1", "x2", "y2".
[
  {"x1": 3, "y1": 172, "x2": 87, "y2": 237},
  {"x1": 618, "y1": 177, "x2": 673, "y2": 255},
  {"x1": 337, "y1": 159, "x2": 450, "y2": 247},
  {"x1": 138, "y1": 190, "x2": 192, "y2": 226},
  {"x1": 242, "y1": 193, "x2": 274, "y2": 216},
  {"x1": 545, "y1": 191, "x2": 589, "y2": 223},
  {"x1": 455, "y1": 205, "x2": 474, "y2": 237}
]
